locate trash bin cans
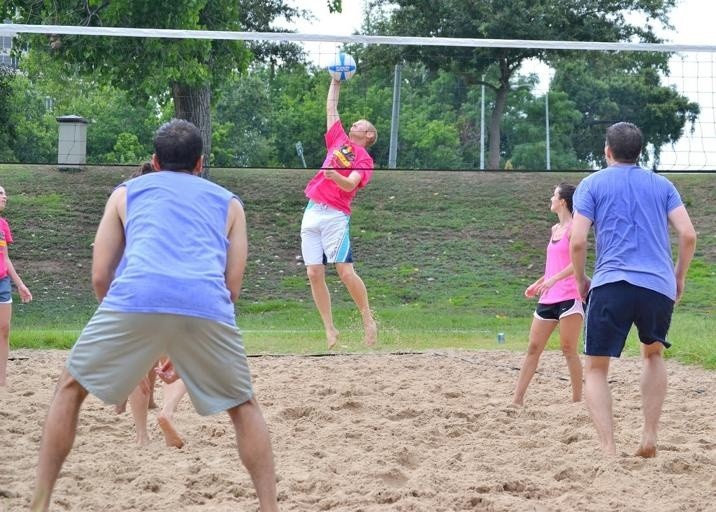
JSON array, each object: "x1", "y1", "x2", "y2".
[{"x1": 56, "y1": 115, "x2": 88, "y2": 164}]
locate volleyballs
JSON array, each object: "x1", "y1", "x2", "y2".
[{"x1": 328, "y1": 52, "x2": 357, "y2": 83}]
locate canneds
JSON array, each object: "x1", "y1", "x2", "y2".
[{"x1": 498, "y1": 332, "x2": 505, "y2": 344}]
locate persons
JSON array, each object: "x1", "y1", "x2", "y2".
[
  {"x1": 114, "y1": 360, "x2": 159, "y2": 415},
  {"x1": 507, "y1": 181, "x2": 588, "y2": 405},
  {"x1": 569, "y1": 123, "x2": 697, "y2": 456},
  {"x1": 111, "y1": 163, "x2": 191, "y2": 448},
  {"x1": 29, "y1": 117, "x2": 279, "y2": 512},
  {"x1": 300, "y1": 78, "x2": 378, "y2": 349},
  {"x1": 0, "y1": 187, "x2": 33, "y2": 395}
]
